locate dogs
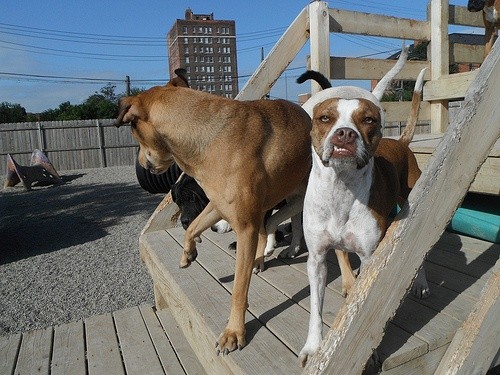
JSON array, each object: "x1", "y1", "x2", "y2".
[
  {"x1": 113, "y1": 37, "x2": 407, "y2": 358},
  {"x1": 297, "y1": 67, "x2": 430, "y2": 368}
]
